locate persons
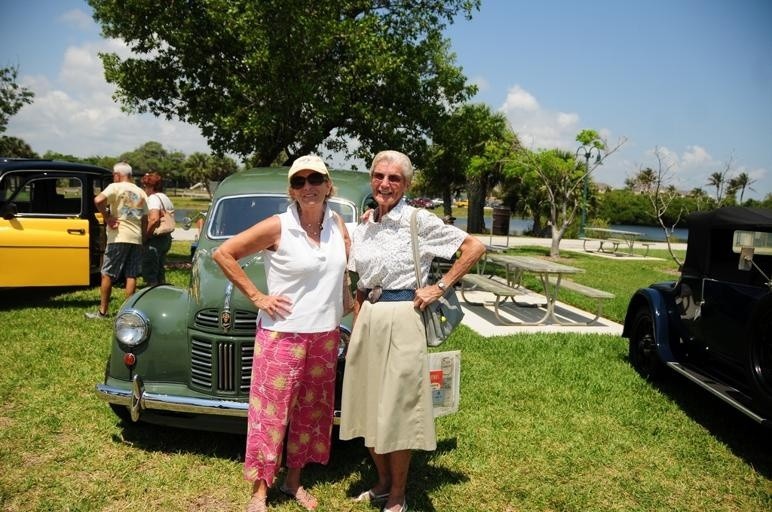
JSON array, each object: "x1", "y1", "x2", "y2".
[
  {"x1": 437, "y1": 215, "x2": 461, "y2": 286},
  {"x1": 85, "y1": 162, "x2": 149, "y2": 319},
  {"x1": 138, "y1": 172, "x2": 176, "y2": 287},
  {"x1": 339, "y1": 151, "x2": 487, "y2": 512},
  {"x1": 215, "y1": 154, "x2": 374, "y2": 512}
]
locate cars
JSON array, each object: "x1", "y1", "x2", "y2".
[
  {"x1": 623, "y1": 205, "x2": 772, "y2": 480},
  {"x1": 0, "y1": 160, "x2": 114, "y2": 312},
  {"x1": 95, "y1": 166, "x2": 376, "y2": 436}
]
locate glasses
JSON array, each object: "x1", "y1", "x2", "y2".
[{"x1": 291, "y1": 172, "x2": 324, "y2": 190}]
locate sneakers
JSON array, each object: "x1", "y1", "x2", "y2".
[{"x1": 85, "y1": 310, "x2": 108, "y2": 320}]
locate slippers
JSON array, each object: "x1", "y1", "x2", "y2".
[
  {"x1": 247, "y1": 494, "x2": 269, "y2": 512},
  {"x1": 352, "y1": 490, "x2": 392, "y2": 504},
  {"x1": 384, "y1": 497, "x2": 410, "y2": 512},
  {"x1": 280, "y1": 486, "x2": 319, "y2": 511}
]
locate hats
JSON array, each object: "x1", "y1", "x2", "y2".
[{"x1": 287, "y1": 154, "x2": 328, "y2": 185}]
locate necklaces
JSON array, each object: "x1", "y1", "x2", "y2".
[{"x1": 298, "y1": 209, "x2": 325, "y2": 236}]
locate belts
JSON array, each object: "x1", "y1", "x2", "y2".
[{"x1": 362, "y1": 285, "x2": 416, "y2": 305}]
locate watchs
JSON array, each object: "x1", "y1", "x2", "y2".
[{"x1": 436, "y1": 279, "x2": 447, "y2": 292}]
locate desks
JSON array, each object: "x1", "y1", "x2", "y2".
[
  {"x1": 487, "y1": 253, "x2": 587, "y2": 324},
  {"x1": 583, "y1": 227, "x2": 642, "y2": 257}
]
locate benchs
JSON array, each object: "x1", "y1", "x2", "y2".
[
  {"x1": 583, "y1": 239, "x2": 624, "y2": 257},
  {"x1": 609, "y1": 239, "x2": 656, "y2": 257},
  {"x1": 459, "y1": 272, "x2": 521, "y2": 326},
  {"x1": 536, "y1": 275, "x2": 616, "y2": 326}
]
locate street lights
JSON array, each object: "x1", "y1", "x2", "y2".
[{"x1": 573, "y1": 146, "x2": 603, "y2": 226}]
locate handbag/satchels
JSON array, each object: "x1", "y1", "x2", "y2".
[
  {"x1": 410, "y1": 208, "x2": 465, "y2": 346},
  {"x1": 152, "y1": 194, "x2": 175, "y2": 237}
]
